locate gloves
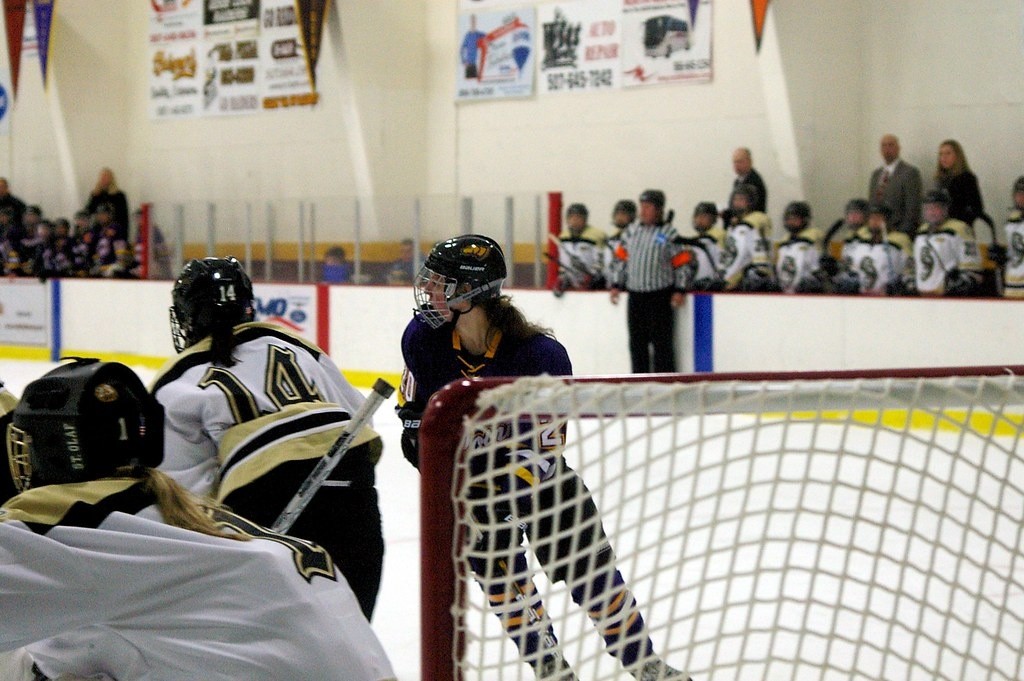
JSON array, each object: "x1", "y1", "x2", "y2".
[
  {"x1": 490, "y1": 446, "x2": 553, "y2": 507},
  {"x1": 397, "y1": 400, "x2": 428, "y2": 470}
]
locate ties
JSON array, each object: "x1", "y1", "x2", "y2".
[{"x1": 877, "y1": 171, "x2": 889, "y2": 202}]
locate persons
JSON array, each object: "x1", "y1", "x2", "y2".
[
  {"x1": 0, "y1": 356, "x2": 398, "y2": 681},
  {"x1": 609, "y1": 189, "x2": 691, "y2": 373},
  {"x1": 145, "y1": 254, "x2": 386, "y2": 621},
  {"x1": 732, "y1": 146, "x2": 767, "y2": 212},
  {"x1": 392, "y1": 240, "x2": 427, "y2": 284},
  {"x1": 323, "y1": 246, "x2": 353, "y2": 283},
  {"x1": 0, "y1": 167, "x2": 172, "y2": 280},
  {"x1": 394, "y1": 234, "x2": 693, "y2": 681},
  {"x1": 553, "y1": 200, "x2": 636, "y2": 298},
  {"x1": 934, "y1": 138, "x2": 983, "y2": 225},
  {"x1": 686, "y1": 183, "x2": 983, "y2": 298},
  {"x1": 460, "y1": 14, "x2": 487, "y2": 77},
  {"x1": 868, "y1": 134, "x2": 922, "y2": 240},
  {"x1": 988, "y1": 175, "x2": 1024, "y2": 298}
]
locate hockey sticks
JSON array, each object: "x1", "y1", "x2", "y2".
[
  {"x1": 271, "y1": 376, "x2": 397, "y2": 536},
  {"x1": 464, "y1": 510, "x2": 578, "y2": 680}
]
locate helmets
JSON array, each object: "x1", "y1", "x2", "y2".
[
  {"x1": 413, "y1": 234, "x2": 507, "y2": 333},
  {"x1": 869, "y1": 205, "x2": 891, "y2": 220},
  {"x1": 640, "y1": 191, "x2": 664, "y2": 209},
  {"x1": 783, "y1": 202, "x2": 808, "y2": 220},
  {"x1": 566, "y1": 204, "x2": 586, "y2": 219},
  {"x1": 922, "y1": 189, "x2": 952, "y2": 209},
  {"x1": 613, "y1": 202, "x2": 636, "y2": 222},
  {"x1": 7, "y1": 356, "x2": 164, "y2": 494},
  {"x1": 844, "y1": 199, "x2": 868, "y2": 218},
  {"x1": 171, "y1": 257, "x2": 258, "y2": 354},
  {"x1": 1013, "y1": 177, "x2": 1024, "y2": 195},
  {"x1": 733, "y1": 184, "x2": 758, "y2": 209},
  {"x1": 693, "y1": 203, "x2": 717, "y2": 218}
]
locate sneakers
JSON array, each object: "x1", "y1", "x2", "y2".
[{"x1": 628, "y1": 651, "x2": 693, "y2": 680}]
